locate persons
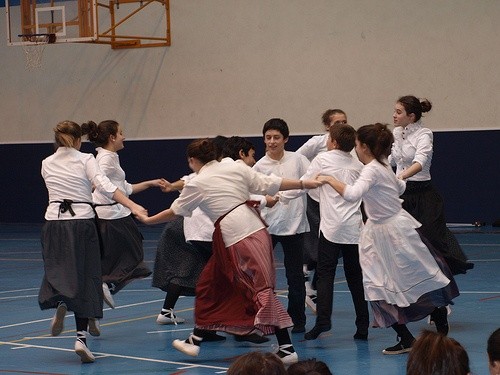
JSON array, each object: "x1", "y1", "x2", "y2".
[
  {"x1": 316, "y1": 122, "x2": 460, "y2": 354},
  {"x1": 387, "y1": 96, "x2": 475, "y2": 323},
  {"x1": 136, "y1": 139, "x2": 323, "y2": 367},
  {"x1": 300, "y1": 123, "x2": 370, "y2": 341},
  {"x1": 486, "y1": 328, "x2": 500, "y2": 375},
  {"x1": 38, "y1": 120, "x2": 147, "y2": 363},
  {"x1": 226, "y1": 351, "x2": 333, "y2": 375},
  {"x1": 152, "y1": 135, "x2": 255, "y2": 326},
  {"x1": 407, "y1": 329, "x2": 472, "y2": 375},
  {"x1": 295, "y1": 109, "x2": 358, "y2": 312},
  {"x1": 81, "y1": 120, "x2": 166, "y2": 337},
  {"x1": 249, "y1": 118, "x2": 311, "y2": 334}
]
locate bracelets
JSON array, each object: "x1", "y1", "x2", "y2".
[{"x1": 300, "y1": 180, "x2": 305, "y2": 190}]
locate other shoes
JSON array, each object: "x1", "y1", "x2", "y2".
[
  {"x1": 157, "y1": 308, "x2": 185, "y2": 325},
  {"x1": 199, "y1": 334, "x2": 227, "y2": 342},
  {"x1": 88, "y1": 316, "x2": 99, "y2": 337},
  {"x1": 234, "y1": 331, "x2": 270, "y2": 344},
  {"x1": 52, "y1": 303, "x2": 67, "y2": 336},
  {"x1": 102, "y1": 282, "x2": 115, "y2": 309},
  {"x1": 270, "y1": 344, "x2": 299, "y2": 363},
  {"x1": 382, "y1": 341, "x2": 416, "y2": 355},
  {"x1": 433, "y1": 308, "x2": 448, "y2": 336},
  {"x1": 75, "y1": 332, "x2": 95, "y2": 362},
  {"x1": 172, "y1": 333, "x2": 203, "y2": 356}
]
